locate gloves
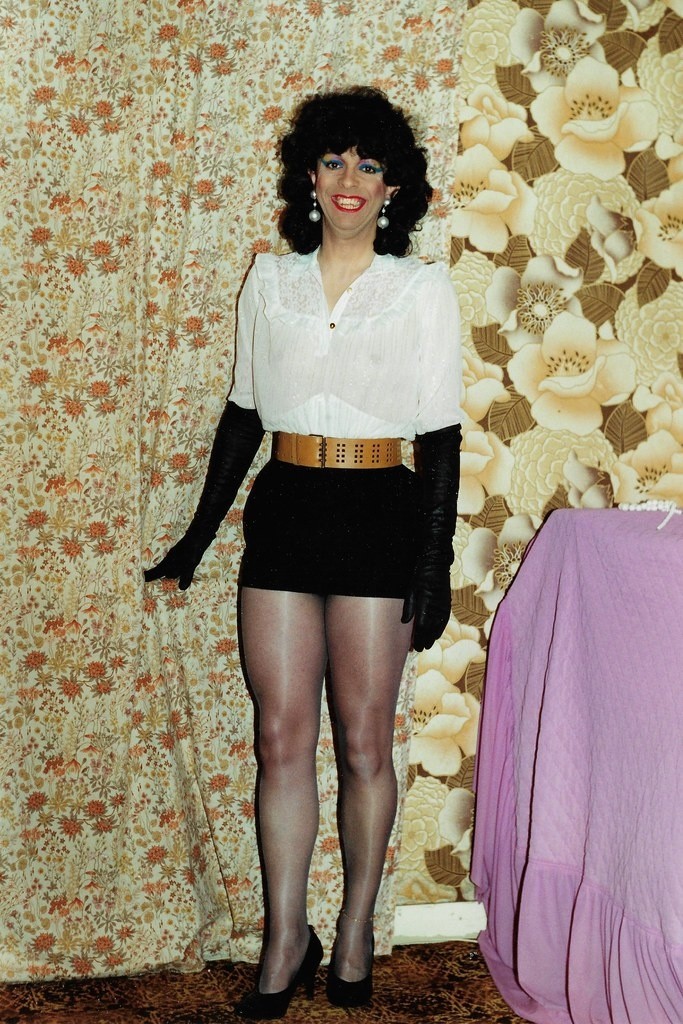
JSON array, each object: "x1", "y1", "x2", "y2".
[
  {"x1": 145, "y1": 400, "x2": 266, "y2": 592},
  {"x1": 396, "y1": 423, "x2": 463, "y2": 651}
]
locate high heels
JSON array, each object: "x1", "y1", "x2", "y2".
[
  {"x1": 326, "y1": 930, "x2": 376, "y2": 1008},
  {"x1": 234, "y1": 924, "x2": 324, "y2": 1020}
]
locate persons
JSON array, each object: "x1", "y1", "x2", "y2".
[{"x1": 144, "y1": 86, "x2": 462, "y2": 1019}]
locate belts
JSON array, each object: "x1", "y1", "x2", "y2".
[{"x1": 275, "y1": 432, "x2": 404, "y2": 469}]
